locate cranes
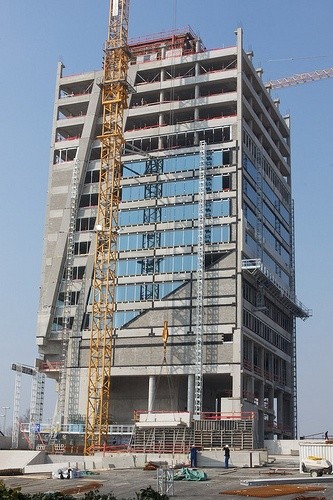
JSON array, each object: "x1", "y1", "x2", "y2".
[
  {"x1": 78, "y1": 0, "x2": 131, "y2": 458},
  {"x1": 258, "y1": 66, "x2": 333, "y2": 92}
]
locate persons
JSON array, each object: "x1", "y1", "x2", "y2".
[
  {"x1": 190, "y1": 445, "x2": 197, "y2": 467},
  {"x1": 223, "y1": 444, "x2": 230, "y2": 469},
  {"x1": 324, "y1": 431, "x2": 329, "y2": 440}
]
